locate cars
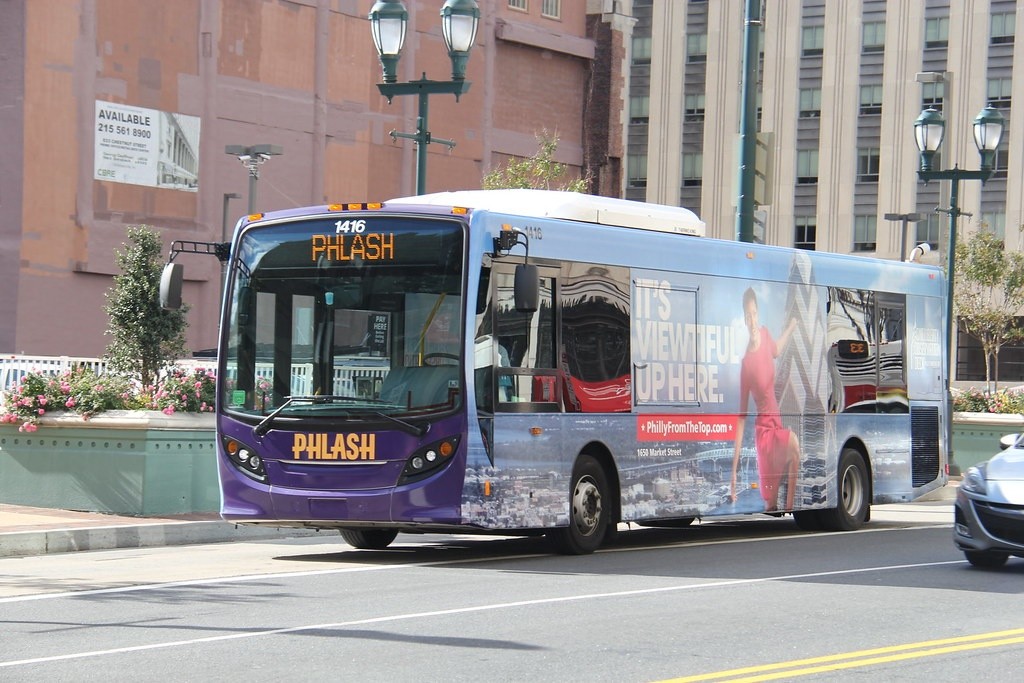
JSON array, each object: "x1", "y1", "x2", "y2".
[{"x1": 955, "y1": 430, "x2": 1024, "y2": 566}]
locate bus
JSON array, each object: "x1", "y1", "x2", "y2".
[{"x1": 162, "y1": 188, "x2": 948, "y2": 551}]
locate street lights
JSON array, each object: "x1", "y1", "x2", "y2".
[
  {"x1": 223, "y1": 142, "x2": 283, "y2": 212},
  {"x1": 915, "y1": 104, "x2": 1006, "y2": 477},
  {"x1": 367, "y1": 0, "x2": 483, "y2": 195}
]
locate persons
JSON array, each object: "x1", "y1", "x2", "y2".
[
  {"x1": 727, "y1": 284, "x2": 805, "y2": 514},
  {"x1": 451, "y1": 313, "x2": 515, "y2": 401}
]
